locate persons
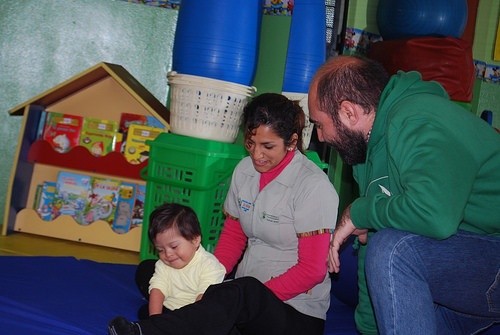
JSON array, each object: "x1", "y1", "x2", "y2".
[
  {"x1": 138, "y1": 202, "x2": 226, "y2": 319},
  {"x1": 108, "y1": 93, "x2": 340, "y2": 335},
  {"x1": 307, "y1": 56, "x2": 500, "y2": 335}
]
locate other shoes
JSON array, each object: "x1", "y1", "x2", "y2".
[{"x1": 108, "y1": 317, "x2": 140, "y2": 335}]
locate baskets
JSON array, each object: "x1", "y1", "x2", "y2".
[
  {"x1": 283, "y1": 91, "x2": 315, "y2": 150},
  {"x1": 165, "y1": 71, "x2": 259, "y2": 141}
]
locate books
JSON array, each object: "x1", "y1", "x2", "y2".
[
  {"x1": 33, "y1": 170, "x2": 146, "y2": 234},
  {"x1": 38, "y1": 112, "x2": 168, "y2": 166}
]
